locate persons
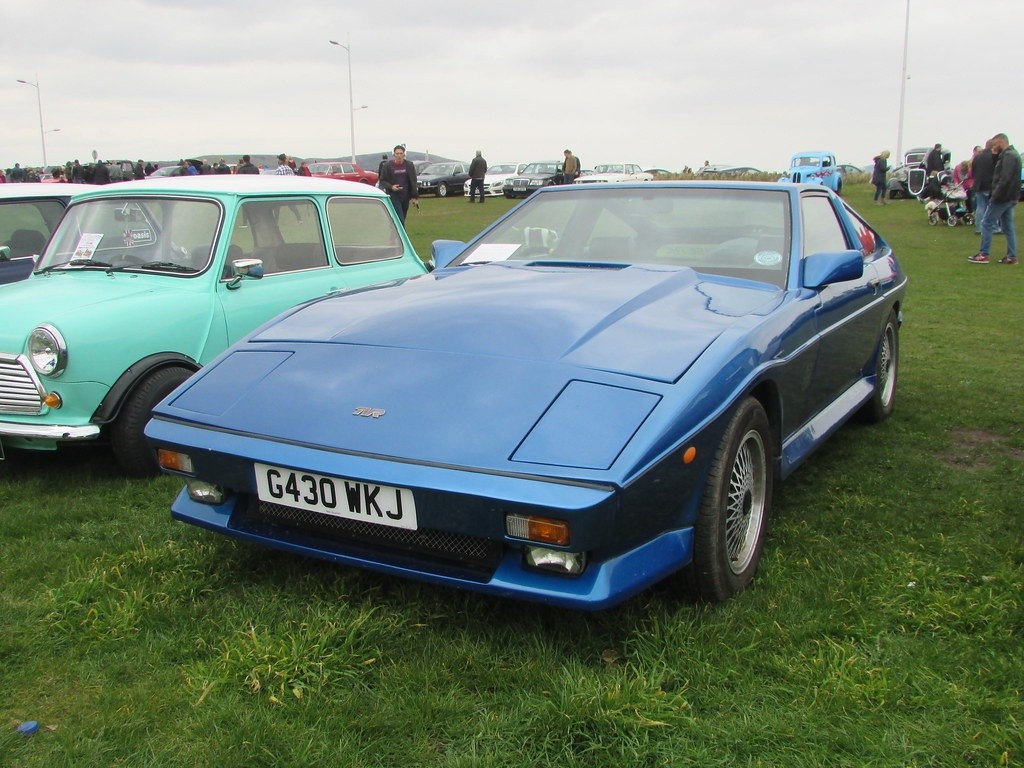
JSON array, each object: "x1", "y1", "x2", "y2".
[
  {"x1": 274, "y1": 154, "x2": 303, "y2": 224},
  {"x1": 468, "y1": 150, "x2": 487, "y2": 203},
  {"x1": 199, "y1": 158, "x2": 231, "y2": 174},
  {"x1": 378, "y1": 155, "x2": 388, "y2": 192},
  {"x1": 233, "y1": 159, "x2": 244, "y2": 173},
  {"x1": 380, "y1": 146, "x2": 418, "y2": 225},
  {"x1": 971, "y1": 139, "x2": 1004, "y2": 235},
  {"x1": 954, "y1": 146, "x2": 983, "y2": 213},
  {"x1": 238, "y1": 155, "x2": 260, "y2": 227},
  {"x1": 871, "y1": 151, "x2": 891, "y2": 205},
  {"x1": 917, "y1": 144, "x2": 944, "y2": 203},
  {"x1": 683, "y1": 166, "x2": 692, "y2": 174},
  {"x1": 297, "y1": 161, "x2": 312, "y2": 176},
  {"x1": 0, "y1": 163, "x2": 44, "y2": 183},
  {"x1": 52, "y1": 159, "x2": 123, "y2": 184},
  {"x1": 288, "y1": 159, "x2": 296, "y2": 170},
  {"x1": 562, "y1": 150, "x2": 581, "y2": 184},
  {"x1": 704, "y1": 161, "x2": 710, "y2": 167},
  {"x1": 134, "y1": 159, "x2": 159, "y2": 180},
  {"x1": 967, "y1": 134, "x2": 1022, "y2": 264}
]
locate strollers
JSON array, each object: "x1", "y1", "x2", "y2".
[{"x1": 924, "y1": 170, "x2": 976, "y2": 227}]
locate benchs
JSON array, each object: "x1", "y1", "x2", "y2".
[{"x1": 275, "y1": 242, "x2": 401, "y2": 270}]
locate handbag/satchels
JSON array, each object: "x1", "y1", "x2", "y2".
[{"x1": 869, "y1": 177, "x2": 876, "y2": 184}]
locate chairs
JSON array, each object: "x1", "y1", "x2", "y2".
[
  {"x1": 192, "y1": 244, "x2": 243, "y2": 268},
  {"x1": 10, "y1": 229, "x2": 45, "y2": 257}
]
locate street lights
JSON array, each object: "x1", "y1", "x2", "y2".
[
  {"x1": 16, "y1": 79, "x2": 63, "y2": 169},
  {"x1": 329, "y1": 31, "x2": 369, "y2": 163}
]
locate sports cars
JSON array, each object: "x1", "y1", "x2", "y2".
[{"x1": 141, "y1": 177, "x2": 909, "y2": 613}]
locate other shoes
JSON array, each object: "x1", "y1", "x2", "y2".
[
  {"x1": 998, "y1": 256, "x2": 1018, "y2": 264},
  {"x1": 967, "y1": 253, "x2": 989, "y2": 264},
  {"x1": 874, "y1": 201, "x2": 878, "y2": 206},
  {"x1": 880, "y1": 201, "x2": 889, "y2": 205}
]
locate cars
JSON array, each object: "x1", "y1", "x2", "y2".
[
  {"x1": 886, "y1": 148, "x2": 952, "y2": 200},
  {"x1": 307, "y1": 162, "x2": 378, "y2": 187},
  {"x1": 145, "y1": 166, "x2": 183, "y2": 177},
  {"x1": 1019, "y1": 153, "x2": 1024, "y2": 203},
  {"x1": 0, "y1": 175, "x2": 435, "y2": 484},
  {"x1": 836, "y1": 163, "x2": 864, "y2": 175},
  {"x1": 777, "y1": 151, "x2": 842, "y2": 195},
  {"x1": 702, "y1": 165, "x2": 763, "y2": 177},
  {"x1": 410, "y1": 159, "x2": 674, "y2": 199},
  {"x1": 0, "y1": 182, "x2": 189, "y2": 286}
]
